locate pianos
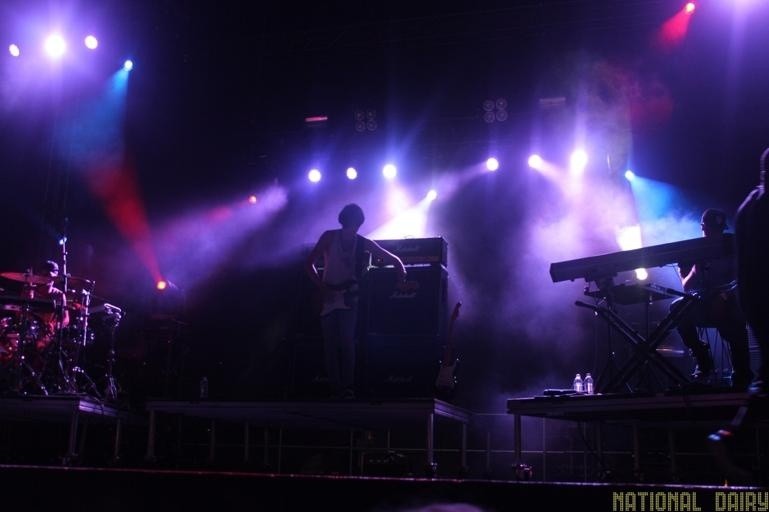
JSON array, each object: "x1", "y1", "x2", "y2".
[{"x1": 549, "y1": 233, "x2": 737, "y2": 283}]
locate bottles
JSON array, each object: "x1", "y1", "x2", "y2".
[
  {"x1": 572, "y1": 374, "x2": 583, "y2": 394},
  {"x1": 583, "y1": 373, "x2": 594, "y2": 394}
]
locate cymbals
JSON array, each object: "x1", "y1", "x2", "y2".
[
  {"x1": 4, "y1": 296, "x2": 50, "y2": 309},
  {"x1": 1, "y1": 272, "x2": 50, "y2": 284}
]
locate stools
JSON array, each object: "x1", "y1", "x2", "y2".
[{"x1": 696, "y1": 321, "x2": 736, "y2": 385}]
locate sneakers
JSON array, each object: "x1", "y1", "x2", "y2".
[
  {"x1": 731, "y1": 368, "x2": 754, "y2": 393},
  {"x1": 692, "y1": 349, "x2": 712, "y2": 381}
]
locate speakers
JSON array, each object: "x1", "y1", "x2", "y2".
[{"x1": 296, "y1": 240, "x2": 448, "y2": 401}]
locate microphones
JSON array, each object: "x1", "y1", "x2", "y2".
[{"x1": 59, "y1": 237, "x2": 68, "y2": 244}]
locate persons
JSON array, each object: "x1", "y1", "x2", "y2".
[
  {"x1": 733, "y1": 147, "x2": 769, "y2": 393},
  {"x1": 306, "y1": 202, "x2": 408, "y2": 401},
  {"x1": 667, "y1": 206, "x2": 756, "y2": 395},
  {"x1": 1, "y1": 259, "x2": 70, "y2": 363}
]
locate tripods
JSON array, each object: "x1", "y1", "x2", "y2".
[{"x1": 0, "y1": 245, "x2": 119, "y2": 401}]
